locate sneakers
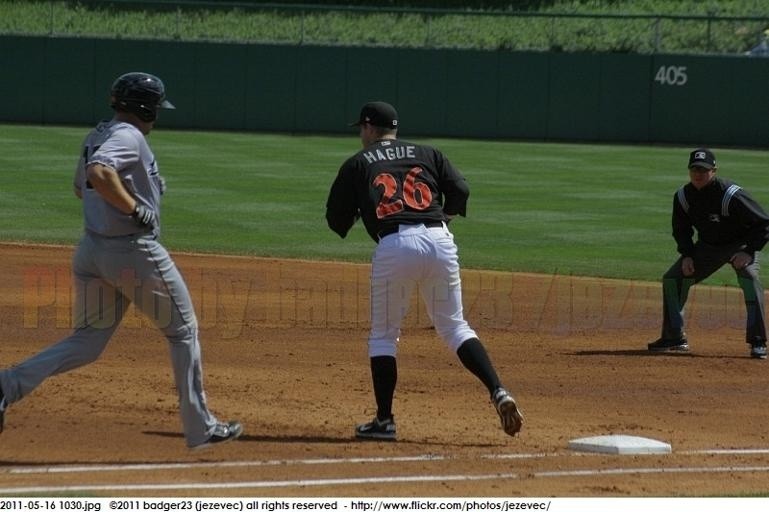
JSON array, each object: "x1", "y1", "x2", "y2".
[
  {"x1": 490, "y1": 387, "x2": 523, "y2": 436},
  {"x1": 751, "y1": 342, "x2": 767, "y2": 358},
  {"x1": 207, "y1": 421, "x2": 241, "y2": 443},
  {"x1": 0, "y1": 386, "x2": 8, "y2": 433},
  {"x1": 355, "y1": 417, "x2": 397, "y2": 438},
  {"x1": 648, "y1": 338, "x2": 688, "y2": 351}
]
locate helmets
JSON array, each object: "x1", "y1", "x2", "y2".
[{"x1": 109, "y1": 71, "x2": 176, "y2": 111}]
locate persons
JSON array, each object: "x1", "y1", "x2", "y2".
[
  {"x1": 323, "y1": 100, "x2": 524, "y2": 441},
  {"x1": 2, "y1": 70, "x2": 244, "y2": 451},
  {"x1": 645, "y1": 147, "x2": 769, "y2": 363}
]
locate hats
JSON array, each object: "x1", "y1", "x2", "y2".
[
  {"x1": 688, "y1": 148, "x2": 715, "y2": 170},
  {"x1": 349, "y1": 102, "x2": 399, "y2": 129}
]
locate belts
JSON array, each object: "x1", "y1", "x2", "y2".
[{"x1": 379, "y1": 220, "x2": 443, "y2": 238}]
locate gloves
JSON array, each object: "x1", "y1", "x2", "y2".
[
  {"x1": 136, "y1": 207, "x2": 154, "y2": 225},
  {"x1": 159, "y1": 178, "x2": 166, "y2": 195}
]
